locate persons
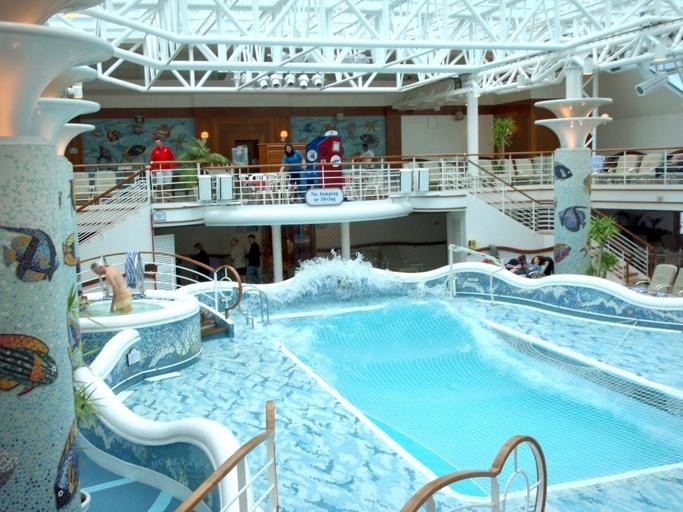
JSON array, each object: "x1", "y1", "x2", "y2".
[
  {"x1": 277, "y1": 144, "x2": 307, "y2": 202},
  {"x1": 228, "y1": 238, "x2": 246, "y2": 284},
  {"x1": 243, "y1": 234, "x2": 261, "y2": 283},
  {"x1": 193, "y1": 242, "x2": 210, "y2": 282},
  {"x1": 148, "y1": 136, "x2": 176, "y2": 202},
  {"x1": 350, "y1": 144, "x2": 375, "y2": 168},
  {"x1": 75, "y1": 289, "x2": 90, "y2": 317},
  {"x1": 89, "y1": 261, "x2": 132, "y2": 314},
  {"x1": 505, "y1": 255, "x2": 543, "y2": 279}
]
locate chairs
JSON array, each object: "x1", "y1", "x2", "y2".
[
  {"x1": 634, "y1": 263, "x2": 683, "y2": 297},
  {"x1": 72, "y1": 171, "x2": 123, "y2": 205},
  {"x1": 239, "y1": 169, "x2": 383, "y2": 206},
  {"x1": 402, "y1": 152, "x2": 682, "y2": 184}
]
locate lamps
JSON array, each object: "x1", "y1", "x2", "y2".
[
  {"x1": 253, "y1": 72, "x2": 323, "y2": 90},
  {"x1": 633, "y1": 68, "x2": 673, "y2": 97}
]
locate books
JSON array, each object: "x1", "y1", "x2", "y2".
[{"x1": 518, "y1": 254, "x2": 527, "y2": 266}]
